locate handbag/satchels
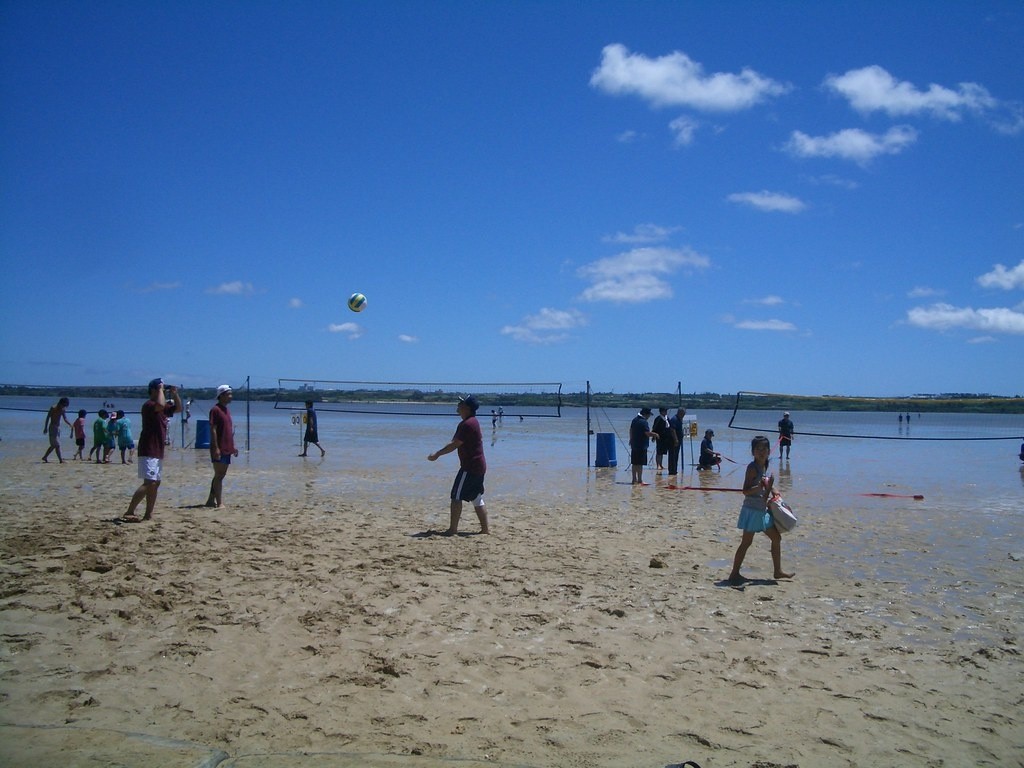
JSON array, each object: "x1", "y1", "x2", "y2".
[{"x1": 767, "y1": 494, "x2": 797, "y2": 531}]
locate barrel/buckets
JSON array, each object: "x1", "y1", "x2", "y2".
[
  {"x1": 195, "y1": 420, "x2": 211, "y2": 448},
  {"x1": 595, "y1": 432, "x2": 618, "y2": 467}
]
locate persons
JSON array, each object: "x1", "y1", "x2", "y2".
[
  {"x1": 166, "y1": 399, "x2": 174, "y2": 446},
  {"x1": 728, "y1": 436, "x2": 796, "y2": 583},
  {"x1": 499, "y1": 406, "x2": 503, "y2": 418},
  {"x1": 906, "y1": 413, "x2": 910, "y2": 423},
  {"x1": 299, "y1": 400, "x2": 325, "y2": 457},
  {"x1": 651, "y1": 406, "x2": 671, "y2": 470},
  {"x1": 88, "y1": 409, "x2": 135, "y2": 464},
  {"x1": 205, "y1": 384, "x2": 239, "y2": 508},
  {"x1": 666, "y1": 407, "x2": 686, "y2": 475},
  {"x1": 42, "y1": 397, "x2": 72, "y2": 463},
  {"x1": 697, "y1": 429, "x2": 722, "y2": 470},
  {"x1": 124, "y1": 378, "x2": 183, "y2": 521},
  {"x1": 629, "y1": 408, "x2": 660, "y2": 485},
  {"x1": 103, "y1": 400, "x2": 114, "y2": 408},
  {"x1": 899, "y1": 413, "x2": 903, "y2": 423},
  {"x1": 185, "y1": 400, "x2": 194, "y2": 423},
  {"x1": 491, "y1": 410, "x2": 498, "y2": 429},
  {"x1": 70, "y1": 409, "x2": 87, "y2": 459},
  {"x1": 778, "y1": 411, "x2": 794, "y2": 460},
  {"x1": 428, "y1": 395, "x2": 489, "y2": 535}
]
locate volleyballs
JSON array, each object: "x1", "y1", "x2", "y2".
[{"x1": 348, "y1": 293, "x2": 367, "y2": 312}]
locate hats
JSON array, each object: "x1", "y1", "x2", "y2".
[
  {"x1": 659, "y1": 407, "x2": 670, "y2": 412},
  {"x1": 642, "y1": 407, "x2": 653, "y2": 415},
  {"x1": 110, "y1": 411, "x2": 117, "y2": 419},
  {"x1": 784, "y1": 412, "x2": 789, "y2": 415},
  {"x1": 458, "y1": 394, "x2": 479, "y2": 410},
  {"x1": 706, "y1": 429, "x2": 714, "y2": 436},
  {"x1": 149, "y1": 378, "x2": 170, "y2": 389},
  {"x1": 216, "y1": 385, "x2": 231, "y2": 399}
]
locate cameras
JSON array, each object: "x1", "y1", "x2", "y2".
[{"x1": 163, "y1": 384, "x2": 172, "y2": 390}]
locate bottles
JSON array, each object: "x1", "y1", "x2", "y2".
[
  {"x1": 303, "y1": 414, "x2": 307, "y2": 423},
  {"x1": 682, "y1": 422, "x2": 697, "y2": 438}
]
